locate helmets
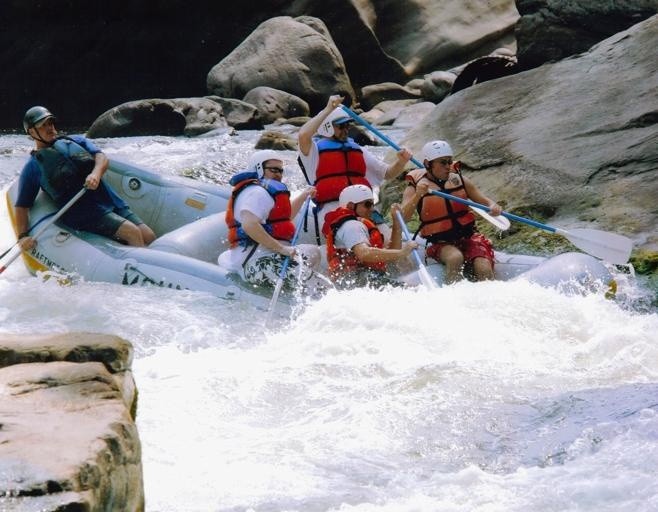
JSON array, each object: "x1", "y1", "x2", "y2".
[
  {"x1": 23, "y1": 106, "x2": 55, "y2": 133},
  {"x1": 422, "y1": 141, "x2": 453, "y2": 163},
  {"x1": 339, "y1": 185, "x2": 375, "y2": 208},
  {"x1": 250, "y1": 150, "x2": 285, "y2": 180},
  {"x1": 317, "y1": 107, "x2": 354, "y2": 137}
]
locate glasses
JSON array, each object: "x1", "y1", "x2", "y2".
[
  {"x1": 433, "y1": 159, "x2": 453, "y2": 165},
  {"x1": 356, "y1": 201, "x2": 374, "y2": 208},
  {"x1": 263, "y1": 167, "x2": 284, "y2": 174},
  {"x1": 334, "y1": 124, "x2": 352, "y2": 131}
]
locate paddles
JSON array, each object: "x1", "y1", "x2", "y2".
[
  {"x1": 426, "y1": 186, "x2": 633, "y2": 266},
  {"x1": 261, "y1": 194, "x2": 310, "y2": 329},
  {"x1": 331, "y1": 102, "x2": 511, "y2": 230},
  {"x1": 391, "y1": 204, "x2": 440, "y2": 293}
]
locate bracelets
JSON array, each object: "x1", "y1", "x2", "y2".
[{"x1": 17, "y1": 233, "x2": 30, "y2": 239}]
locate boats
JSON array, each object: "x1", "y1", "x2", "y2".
[{"x1": 7, "y1": 160, "x2": 617, "y2": 329}]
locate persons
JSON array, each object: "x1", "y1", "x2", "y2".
[
  {"x1": 297, "y1": 94, "x2": 414, "y2": 245},
  {"x1": 13, "y1": 105, "x2": 157, "y2": 251},
  {"x1": 401, "y1": 139, "x2": 502, "y2": 285},
  {"x1": 323, "y1": 181, "x2": 420, "y2": 291},
  {"x1": 224, "y1": 150, "x2": 338, "y2": 302}
]
